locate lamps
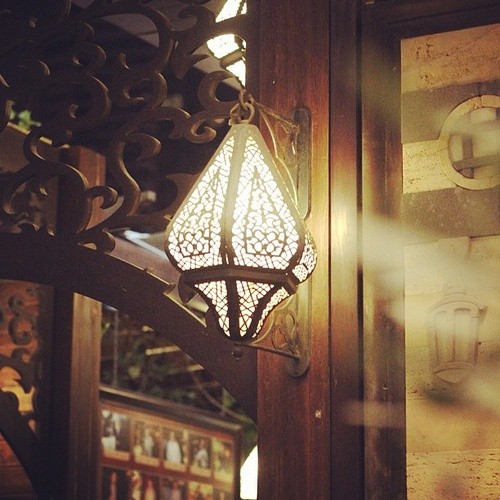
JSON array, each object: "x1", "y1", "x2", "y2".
[
  {"x1": 204, "y1": 0, "x2": 247, "y2": 87},
  {"x1": 162, "y1": 88, "x2": 320, "y2": 378}
]
